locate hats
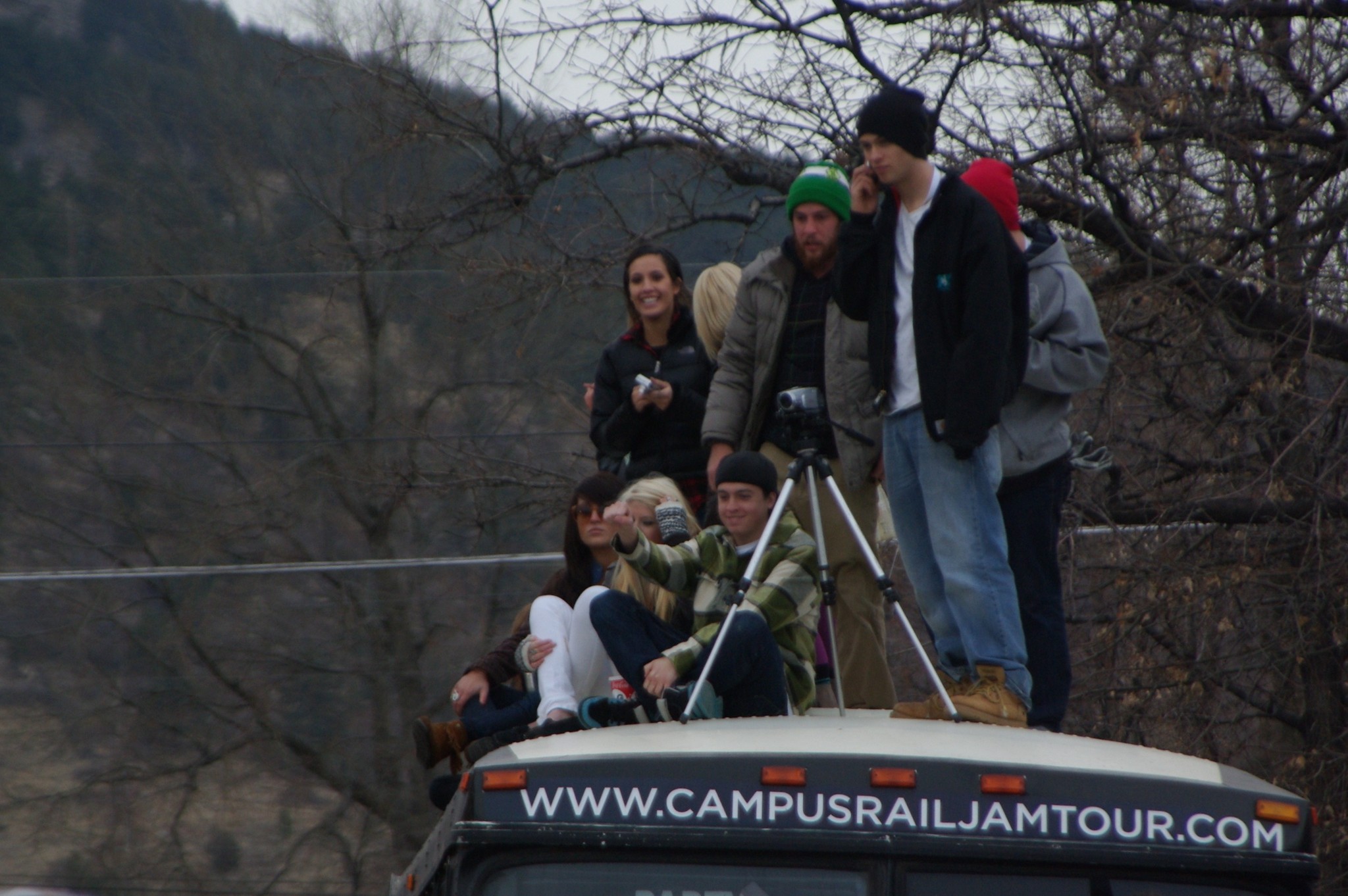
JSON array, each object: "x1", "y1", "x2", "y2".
[
  {"x1": 785, "y1": 159, "x2": 851, "y2": 225},
  {"x1": 714, "y1": 450, "x2": 778, "y2": 495},
  {"x1": 960, "y1": 158, "x2": 1020, "y2": 232},
  {"x1": 856, "y1": 86, "x2": 935, "y2": 162}
]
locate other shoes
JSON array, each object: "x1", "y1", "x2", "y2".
[
  {"x1": 1027, "y1": 711, "x2": 1063, "y2": 733},
  {"x1": 514, "y1": 717, "x2": 582, "y2": 741}
]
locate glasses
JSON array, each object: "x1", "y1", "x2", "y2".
[{"x1": 571, "y1": 499, "x2": 617, "y2": 525}]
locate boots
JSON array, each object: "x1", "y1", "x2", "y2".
[
  {"x1": 412, "y1": 716, "x2": 467, "y2": 769},
  {"x1": 429, "y1": 773, "x2": 461, "y2": 810},
  {"x1": 465, "y1": 732, "x2": 521, "y2": 765}
]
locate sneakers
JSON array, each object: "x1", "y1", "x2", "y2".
[
  {"x1": 664, "y1": 679, "x2": 724, "y2": 719},
  {"x1": 577, "y1": 694, "x2": 661, "y2": 730},
  {"x1": 889, "y1": 667, "x2": 964, "y2": 721},
  {"x1": 949, "y1": 664, "x2": 1027, "y2": 726}
]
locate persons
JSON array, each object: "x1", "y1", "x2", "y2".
[
  {"x1": 413, "y1": 234, "x2": 823, "y2": 812},
  {"x1": 956, "y1": 156, "x2": 1110, "y2": 731},
  {"x1": 702, "y1": 158, "x2": 900, "y2": 711},
  {"x1": 827, "y1": 85, "x2": 1031, "y2": 728}
]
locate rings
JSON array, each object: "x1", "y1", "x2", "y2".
[{"x1": 451, "y1": 691, "x2": 460, "y2": 701}]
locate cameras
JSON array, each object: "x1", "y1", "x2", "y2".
[
  {"x1": 634, "y1": 373, "x2": 661, "y2": 394},
  {"x1": 777, "y1": 384, "x2": 827, "y2": 424}
]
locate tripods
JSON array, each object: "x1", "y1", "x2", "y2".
[{"x1": 676, "y1": 415, "x2": 964, "y2": 725}]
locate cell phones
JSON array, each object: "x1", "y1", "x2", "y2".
[{"x1": 865, "y1": 159, "x2": 879, "y2": 188}]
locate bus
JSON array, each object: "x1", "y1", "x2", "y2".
[{"x1": 395, "y1": 708, "x2": 1326, "y2": 896}]
